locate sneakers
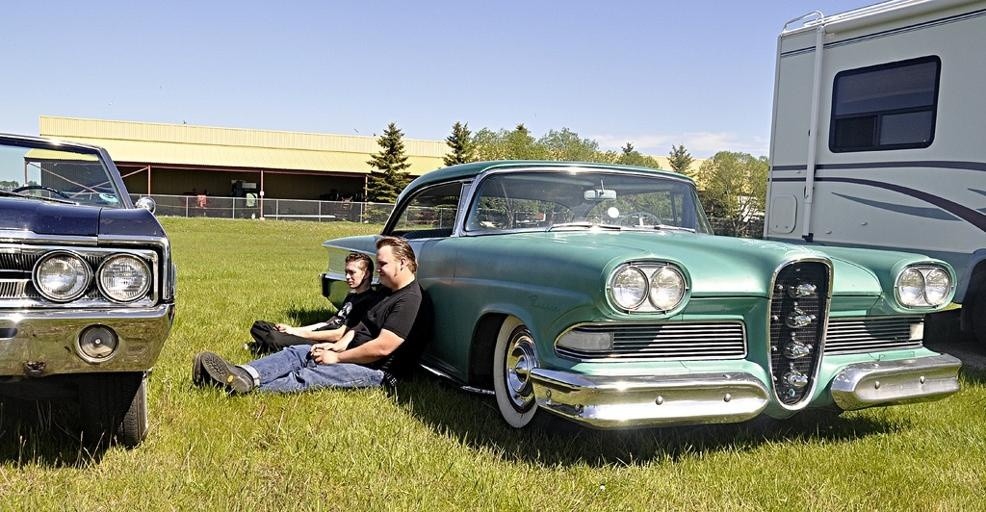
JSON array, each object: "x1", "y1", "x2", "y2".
[{"x1": 191, "y1": 351, "x2": 253, "y2": 394}]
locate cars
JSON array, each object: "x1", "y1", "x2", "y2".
[
  {"x1": 0, "y1": 131, "x2": 173, "y2": 446},
  {"x1": 314, "y1": 159, "x2": 969, "y2": 442}
]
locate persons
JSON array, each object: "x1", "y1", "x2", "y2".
[
  {"x1": 244, "y1": 252, "x2": 381, "y2": 361},
  {"x1": 192, "y1": 236, "x2": 436, "y2": 399}
]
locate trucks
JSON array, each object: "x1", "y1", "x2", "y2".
[{"x1": 756, "y1": 2, "x2": 986, "y2": 361}]
